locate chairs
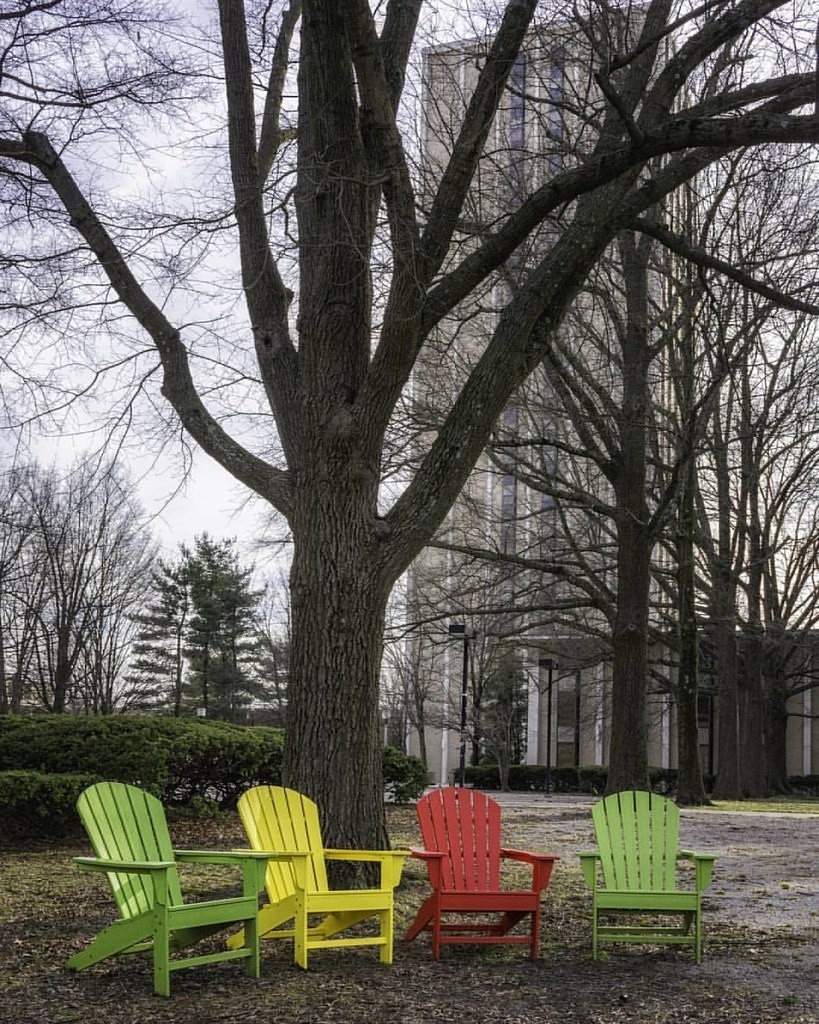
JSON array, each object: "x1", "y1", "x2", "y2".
[
  {"x1": 223, "y1": 784, "x2": 413, "y2": 972},
  {"x1": 62, "y1": 780, "x2": 279, "y2": 997},
  {"x1": 402, "y1": 785, "x2": 561, "y2": 961},
  {"x1": 573, "y1": 790, "x2": 720, "y2": 967}
]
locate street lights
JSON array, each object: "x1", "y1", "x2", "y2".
[
  {"x1": 196, "y1": 707, "x2": 207, "y2": 720},
  {"x1": 538, "y1": 657, "x2": 559, "y2": 769},
  {"x1": 449, "y1": 624, "x2": 475, "y2": 788}
]
